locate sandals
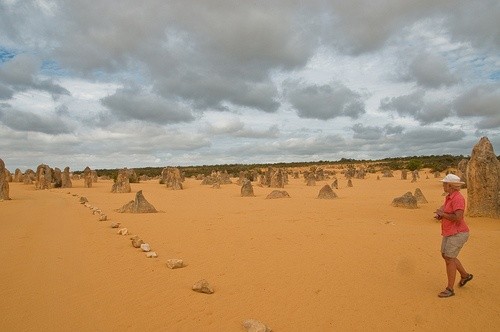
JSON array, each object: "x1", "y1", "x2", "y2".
[
  {"x1": 438, "y1": 288, "x2": 456, "y2": 298},
  {"x1": 458, "y1": 273, "x2": 473, "y2": 287}
]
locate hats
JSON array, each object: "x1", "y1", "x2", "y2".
[{"x1": 437, "y1": 174, "x2": 466, "y2": 184}]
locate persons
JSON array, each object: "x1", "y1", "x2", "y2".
[{"x1": 433, "y1": 174, "x2": 474, "y2": 298}]
